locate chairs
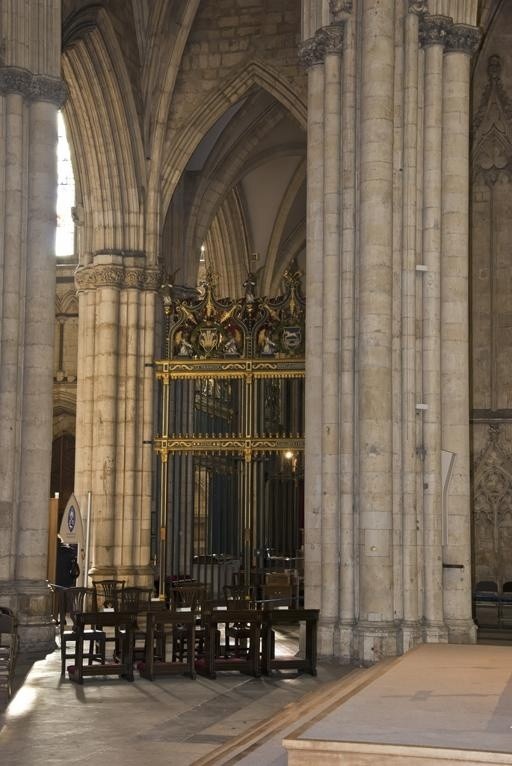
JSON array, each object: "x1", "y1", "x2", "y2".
[
  {"x1": 1, "y1": 606, "x2": 22, "y2": 699},
  {"x1": 47, "y1": 563, "x2": 322, "y2": 685},
  {"x1": 499, "y1": 582, "x2": 511, "y2": 631},
  {"x1": 472, "y1": 580, "x2": 499, "y2": 630}
]
